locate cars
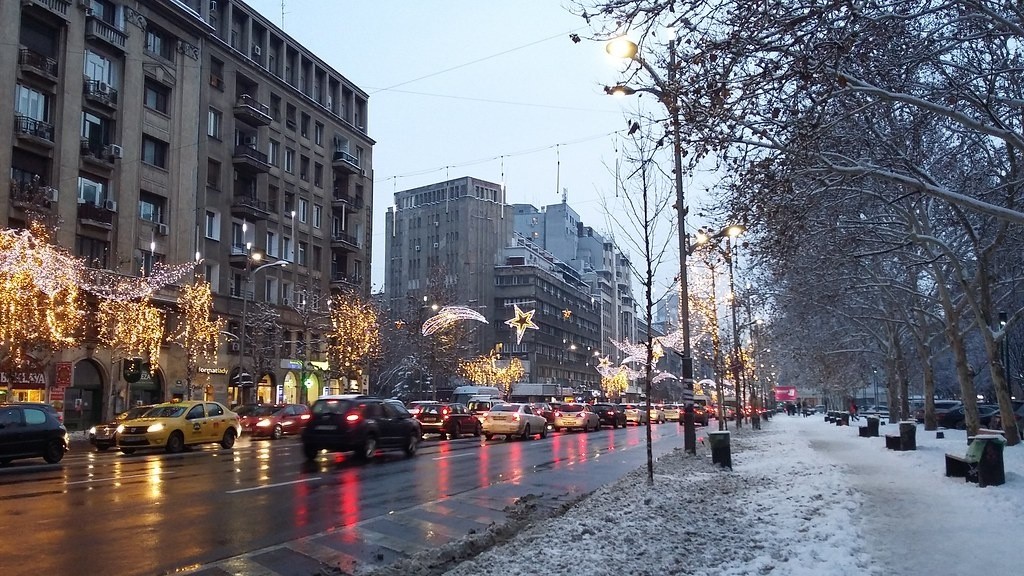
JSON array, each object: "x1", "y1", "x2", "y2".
[
  {"x1": 89, "y1": 404, "x2": 161, "y2": 451},
  {"x1": 531, "y1": 402, "x2": 601, "y2": 433},
  {"x1": 592, "y1": 403, "x2": 626, "y2": 429},
  {"x1": 639, "y1": 403, "x2": 665, "y2": 424},
  {"x1": 116, "y1": 398, "x2": 242, "y2": 454},
  {"x1": 679, "y1": 406, "x2": 709, "y2": 426},
  {"x1": 715, "y1": 406, "x2": 735, "y2": 420},
  {"x1": 231, "y1": 403, "x2": 312, "y2": 440},
  {"x1": 447, "y1": 386, "x2": 548, "y2": 440},
  {"x1": 664, "y1": 404, "x2": 681, "y2": 421},
  {"x1": 857, "y1": 400, "x2": 1024, "y2": 440},
  {"x1": 403, "y1": 401, "x2": 482, "y2": 440}
]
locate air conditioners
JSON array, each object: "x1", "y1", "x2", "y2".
[
  {"x1": 46, "y1": 188, "x2": 58, "y2": 202},
  {"x1": 434, "y1": 242, "x2": 438, "y2": 248},
  {"x1": 244, "y1": 135, "x2": 257, "y2": 146},
  {"x1": 96, "y1": 79, "x2": 110, "y2": 95},
  {"x1": 415, "y1": 245, "x2": 420, "y2": 250},
  {"x1": 103, "y1": 199, "x2": 118, "y2": 212},
  {"x1": 434, "y1": 222, "x2": 439, "y2": 226},
  {"x1": 158, "y1": 223, "x2": 170, "y2": 235},
  {"x1": 109, "y1": 144, "x2": 125, "y2": 159},
  {"x1": 361, "y1": 169, "x2": 368, "y2": 177},
  {"x1": 252, "y1": 45, "x2": 262, "y2": 56}
]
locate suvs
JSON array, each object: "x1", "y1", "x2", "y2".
[
  {"x1": 0, "y1": 402, "x2": 69, "y2": 466},
  {"x1": 618, "y1": 403, "x2": 647, "y2": 426},
  {"x1": 300, "y1": 395, "x2": 423, "y2": 461}
]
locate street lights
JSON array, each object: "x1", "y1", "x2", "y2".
[
  {"x1": 240, "y1": 260, "x2": 289, "y2": 400},
  {"x1": 420, "y1": 296, "x2": 440, "y2": 399},
  {"x1": 605, "y1": 37, "x2": 755, "y2": 454},
  {"x1": 560, "y1": 339, "x2": 577, "y2": 401},
  {"x1": 586, "y1": 349, "x2": 600, "y2": 402}
]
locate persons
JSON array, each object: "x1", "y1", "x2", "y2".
[
  {"x1": 797, "y1": 401, "x2": 807, "y2": 418},
  {"x1": 258, "y1": 396, "x2": 263, "y2": 403},
  {"x1": 786, "y1": 402, "x2": 795, "y2": 416},
  {"x1": 849, "y1": 400, "x2": 859, "y2": 421}
]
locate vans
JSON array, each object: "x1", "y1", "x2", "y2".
[
  {"x1": 721, "y1": 397, "x2": 738, "y2": 417},
  {"x1": 694, "y1": 396, "x2": 714, "y2": 417},
  {"x1": 815, "y1": 405, "x2": 825, "y2": 411}
]
